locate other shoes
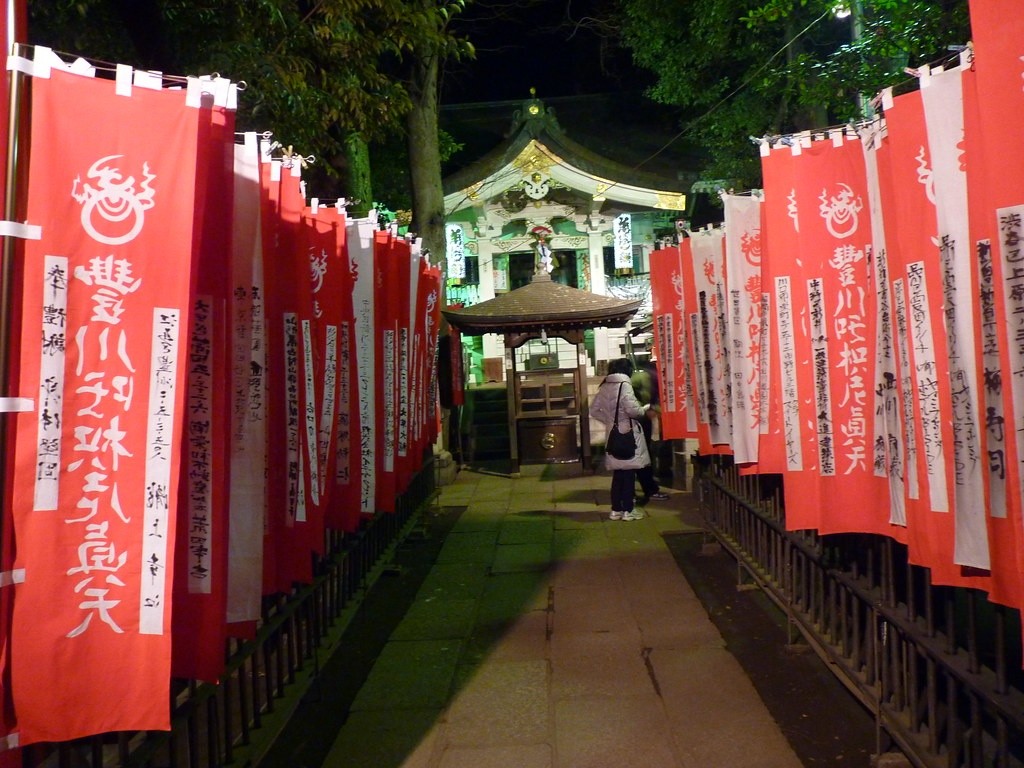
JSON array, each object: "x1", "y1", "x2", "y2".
[{"x1": 650, "y1": 492, "x2": 668, "y2": 500}]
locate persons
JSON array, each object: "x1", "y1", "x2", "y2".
[{"x1": 590, "y1": 359, "x2": 669, "y2": 521}]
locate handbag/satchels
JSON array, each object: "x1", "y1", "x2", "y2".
[{"x1": 605, "y1": 424, "x2": 637, "y2": 460}]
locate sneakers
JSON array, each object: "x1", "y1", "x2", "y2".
[
  {"x1": 609, "y1": 511, "x2": 624, "y2": 520},
  {"x1": 622, "y1": 509, "x2": 643, "y2": 521}
]
